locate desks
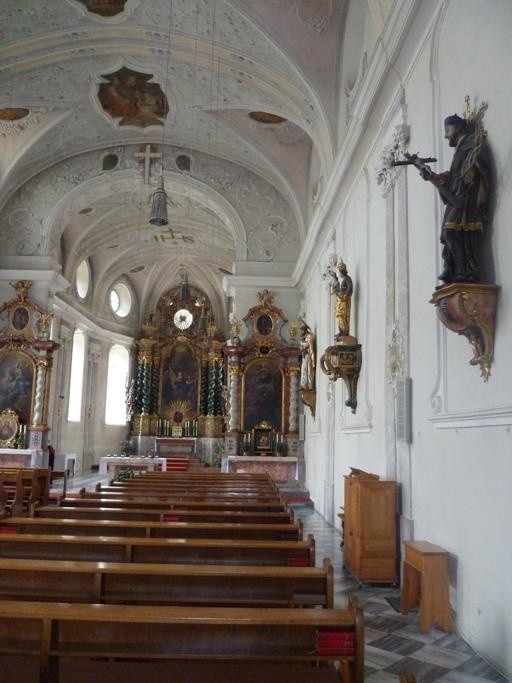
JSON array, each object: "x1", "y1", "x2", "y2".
[{"x1": 98, "y1": 455, "x2": 166, "y2": 481}]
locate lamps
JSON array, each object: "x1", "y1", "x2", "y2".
[{"x1": 149, "y1": 1, "x2": 169, "y2": 226}]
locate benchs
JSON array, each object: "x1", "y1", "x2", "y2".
[{"x1": 1, "y1": 466, "x2": 365, "y2": 682}]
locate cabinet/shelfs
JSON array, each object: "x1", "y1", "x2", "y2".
[{"x1": 341, "y1": 475, "x2": 400, "y2": 589}]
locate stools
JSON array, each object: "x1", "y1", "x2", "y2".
[{"x1": 399, "y1": 542, "x2": 454, "y2": 635}]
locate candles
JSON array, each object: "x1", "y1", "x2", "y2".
[
  {"x1": 24, "y1": 425, "x2": 27, "y2": 435},
  {"x1": 156, "y1": 419, "x2": 170, "y2": 436},
  {"x1": 19, "y1": 424, "x2": 23, "y2": 433},
  {"x1": 244, "y1": 434, "x2": 246, "y2": 443},
  {"x1": 280, "y1": 434, "x2": 283, "y2": 442},
  {"x1": 247, "y1": 433, "x2": 251, "y2": 445},
  {"x1": 184, "y1": 419, "x2": 198, "y2": 436},
  {"x1": 276, "y1": 433, "x2": 279, "y2": 444}
]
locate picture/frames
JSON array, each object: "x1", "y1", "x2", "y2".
[{"x1": 0, "y1": 407, "x2": 19, "y2": 448}]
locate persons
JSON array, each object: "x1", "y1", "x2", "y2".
[
  {"x1": 297, "y1": 324, "x2": 316, "y2": 392},
  {"x1": 0, "y1": 367, "x2": 33, "y2": 420},
  {"x1": 418, "y1": 115, "x2": 493, "y2": 290},
  {"x1": 332, "y1": 262, "x2": 353, "y2": 341},
  {"x1": 162, "y1": 368, "x2": 196, "y2": 409}
]
